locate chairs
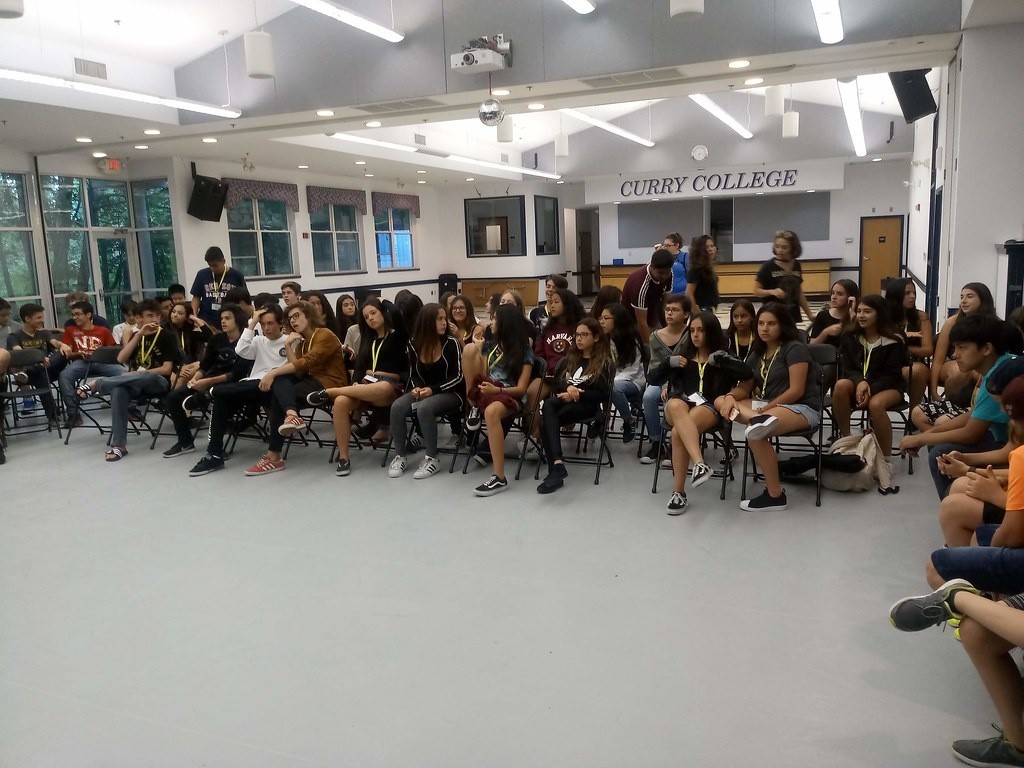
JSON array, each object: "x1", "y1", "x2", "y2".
[{"x1": 0, "y1": 329, "x2": 932, "y2": 507}]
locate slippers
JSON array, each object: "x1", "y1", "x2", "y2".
[{"x1": 75, "y1": 383, "x2": 98, "y2": 399}]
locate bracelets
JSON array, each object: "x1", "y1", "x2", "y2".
[
  {"x1": 757, "y1": 407, "x2": 762, "y2": 413},
  {"x1": 968, "y1": 467, "x2": 976, "y2": 472},
  {"x1": 724, "y1": 393, "x2": 735, "y2": 400},
  {"x1": 500, "y1": 387, "x2": 502, "y2": 392}
]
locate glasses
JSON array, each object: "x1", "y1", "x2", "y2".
[
  {"x1": 662, "y1": 244, "x2": 675, "y2": 248},
  {"x1": 287, "y1": 312, "x2": 299, "y2": 320},
  {"x1": 453, "y1": 305, "x2": 466, "y2": 312},
  {"x1": 72, "y1": 312, "x2": 86, "y2": 316},
  {"x1": 490, "y1": 313, "x2": 497, "y2": 320},
  {"x1": 664, "y1": 307, "x2": 684, "y2": 313},
  {"x1": 598, "y1": 317, "x2": 613, "y2": 323},
  {"x1": 574, "y1": 331, "x2": 593, "y2": 339}
]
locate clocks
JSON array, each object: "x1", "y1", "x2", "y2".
[{"x1": 691, "y1": 145, "x2": 710, "y2": 164}]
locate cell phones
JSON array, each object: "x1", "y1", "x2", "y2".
[{"x1": 728, "y1": 407, "x2": 740, "y2": 421}]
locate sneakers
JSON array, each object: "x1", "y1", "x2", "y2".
[
  {"x1": 279, "y1": 414, "x2": 307, "y2": 436},
  {"x1": 306, "y1": 388, "x2": 331, "y2": 405},
  {"x1": 447, "y1": 402, "x2": 846, "y2": 517},
  {"x1": 3, "y1": 394, "x2": 166, "y2": 427},
  {"x1": 889, "y1": 578, "x2": 975, "y2": 632},
  {"x1": 183, "y1": 387, "x2": 214, "y2": 410},
  {"x1": 950, "y1": 729, "x2": 1024, "y2": 768},
  {"x1": 102, "y1": 433, "x2": 439, "y2": 482},
  {"x1": 14, "y1": 372, "x2": 29, "y2": 383}
]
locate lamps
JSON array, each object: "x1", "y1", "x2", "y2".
[
  {"x1": 910, "y1": 159, "x2": 930, "y2": 169},
  {"x1": 326, "y1": 133, "x2": 418, "y2": 153},
  {"x1": 242, "y1": 159, "x2": 255, "y2": 172},
  {"x1": 556, "y1": 117, "x2": 569, "y2": 157},
  {"x1": 903, "y1": 179, "x2": 921, "y2": 188},
  {"x1": 497, "y1": 116, "x2": 512, "y2": 141},
  {"x1": 0, "y1": 1, "x2": 23, "y2": 17},
  {"x1": 764, "y1": 85, "x2": 799, "y2": 139},
  {"x1": 244, "y1": 1, "x2": 275, "y2": 79},
  {"x1": 559, "y1": 101, "x2": 655, "y2": 148},
  {"x1": 563, "y1": 0, "x2": 599, "y2": 15},
  {"x1": 0, "y1": 31, "x2": 243, "y2": 119},
  {"x1": 446, "y1": 132, "x2": 562, "y2": 180},
  {"x1": 810, "y1": 0, "x2": 844, "y2": 44},
  {"x1": 670, "y1": 0, "x2": 704, "y2": 20},
  {"x1": 837, "y1": 76, "x2": 867, "y2": 157},
  {"x1": 287, "y1": 0, "x2": 405, "y2": 43},
  {"x1": 688, "y1": 90, "x2": 753, "y2": 140}
]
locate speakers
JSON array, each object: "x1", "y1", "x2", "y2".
[
  {"x1": 188, "y1": 175, "x2": 229, "y2": 222},
  {"x1": 888, "y1": 70, "x2": 938, "y2": 124}
]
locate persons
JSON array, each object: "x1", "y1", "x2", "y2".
[
  {"x1": 809, "y1": 279, "x2": 858, "y2": 344},
  {"x1": 588, "y1": 285, "x2": 623, "y2": 312},
  {"x1": 0, "y1": 247, "x2": 535, "y2": 497},
  {"x1": 621, "y1": 249, "x2": 674, "y2": 381},
  {"x1": 640, "y1": 295, "x2": 691, "y2": 468},
  {"x1": 833, "y1": 295, "x2": 905, "y2": 463},
  {"x1": 884, "y1": 279, "x2": 1024, "y2": 768},
  {"x1": 518, "y1": 290, "x2": 586, "y2": 461},
  {"x1": 648, "y1": 310, "x2": 755, "y2": 516},
  {"x1": 527, "y1": 274, "x2": 567, "y2": 332},
  {"x1": 754, "y1": 231, "x2": 817, "y2": 324},
  {"x1": 588, "y1": 304, "x2": 647, "y2": 444},
  {"x1": 686, "y1": 234, "x2": 719, "y2": 316},
  {"x1": 714, "y1": 301, "x2": 820, "y2": 513},
  {"x1": 655, "y1": 232, "x2": 689, "y2": 295},
  {"x1": 718, "y1": 299, "x2": 755, "y2": 470},
  {"x1": 536, "y1": 318, "x2": 612, "y2": 494}
]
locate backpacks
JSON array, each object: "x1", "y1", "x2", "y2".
[{"x1": 822, "y1": 427, "x2": 896, "y2": 493}]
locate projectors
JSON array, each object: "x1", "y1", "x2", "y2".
[{"x1": 451, "y1": 50, "x2": 506, "y2": 75}]
